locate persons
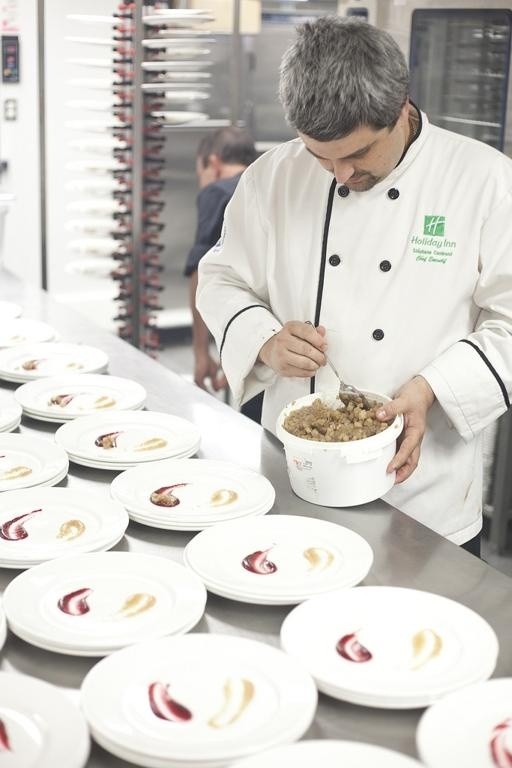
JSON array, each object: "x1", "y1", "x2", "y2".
[
  {"x1": 180, "y1": 123, "x2": 260, "y2": 405},
  {"x1": 193, "y1": 11, "x2": 512, "y2": 561}
]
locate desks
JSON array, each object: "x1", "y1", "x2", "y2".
[{"x1": 2, "y1": 261, "x2": 512, "y2": 768}]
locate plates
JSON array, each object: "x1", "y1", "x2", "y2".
[
  {"x1": 56, "y1": 410, "x2": 202, "y2": 472},
  {"x1": 277, "y1": 586, "x2": 499, "y2": 711},
  {"x1": 0, "y1": 670, "x2": 93, "y2": 767},
  {"x1": 181, "y1": 515, "x2": 375, "y2": 607},
  {"x1": 0, "y1": 433, "x2": 70, "y2": 492},
  {"x1": 0, "y1": 343, "x2": 111, "y2": 384},
  {"x1": 3, "y1": 552, "x2": 209, "y2": 659},
  {"x1": 60, "y1": 8, "x2": 127, "y2": 340},
  {"x1": 13, "y1": 374, "x2": 149, "y2": 423},
  {"x1": 414, "y1": 677, "x2": 512, "y2": 766},
  {"x1": 234, "y1": 740, "x2": 422, "y2": 768},
  {"x1": 110, "y1": 458, "x2": 276, "y2": 533},
  {"x1": 0, "y1": 395, "x2": 25, "y2": 433},
  {"x1": 144, "y1": 4, "x2": 215, "y2": 122},
  {"x1": 75, "y1": 633, "x2": 319, "y2": 766},
  {"x1": 0, "y1": 486, "x2": 131, "y2": 570}
]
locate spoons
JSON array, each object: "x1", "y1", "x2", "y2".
[{"x1": 305, "y1": 319, "x2": 374, "y2": 410}]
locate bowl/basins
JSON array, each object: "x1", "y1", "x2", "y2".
[{"x1": 276, "y1": 389, "x2": 405, "y2": 509}]
